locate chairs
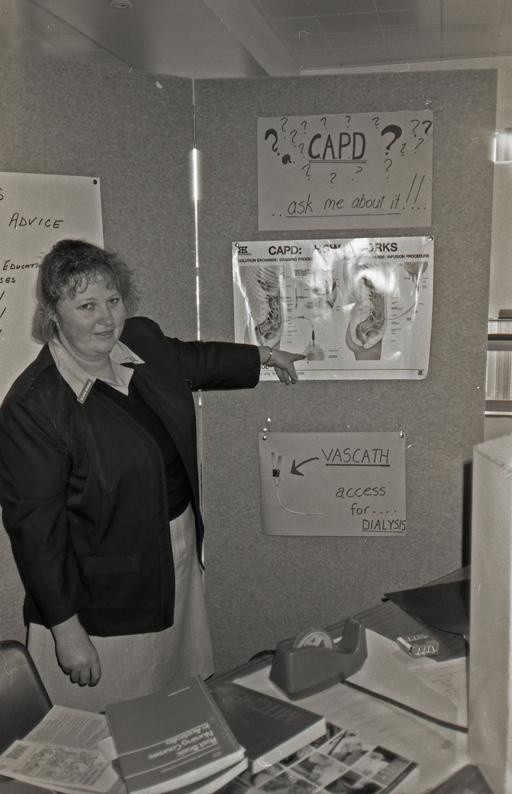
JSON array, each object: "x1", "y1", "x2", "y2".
[{"x1": 1, "y1": 639, "x2": 53, "y2": 754}]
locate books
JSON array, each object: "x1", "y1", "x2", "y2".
[{"x1": 2, "y1": 676, "x2": 421, "y2": 794}]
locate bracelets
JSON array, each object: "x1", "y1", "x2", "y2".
[{"x1": 262, "y1": 346, "x2": 273, "y2": 369}]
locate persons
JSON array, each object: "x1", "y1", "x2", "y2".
[{"x1": 2, "y1": 239, "x2": 306, "y2": 715}]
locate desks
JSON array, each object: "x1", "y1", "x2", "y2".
[{"x1": 207, "y1": 563, "x2": 495, "y2": 794}]
